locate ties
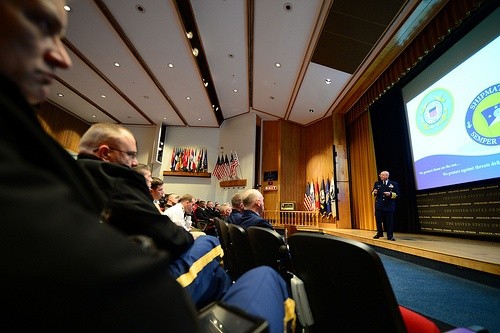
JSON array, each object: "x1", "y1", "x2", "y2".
[{"x1": 384, "y1": 181, "x2": 386, "y2": 185}]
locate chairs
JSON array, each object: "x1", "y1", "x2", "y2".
[{"x1": 192, "y1": 211, "x2": 490, "y2": 333}]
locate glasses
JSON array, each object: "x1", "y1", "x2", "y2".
[
  {"x1": 379, "y1": 175, "x2": 385, "y2": 176},
  {"x1": 93, "y1": 148, "x2": 137, "y2": 159}
]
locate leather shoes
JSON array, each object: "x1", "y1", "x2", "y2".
[
  {"x1": 373, "y1": 235, "x2": 383, "y2": 239},
  {"x1": 387, "y1": 237, "x2": 395, "y2": 241}
]
